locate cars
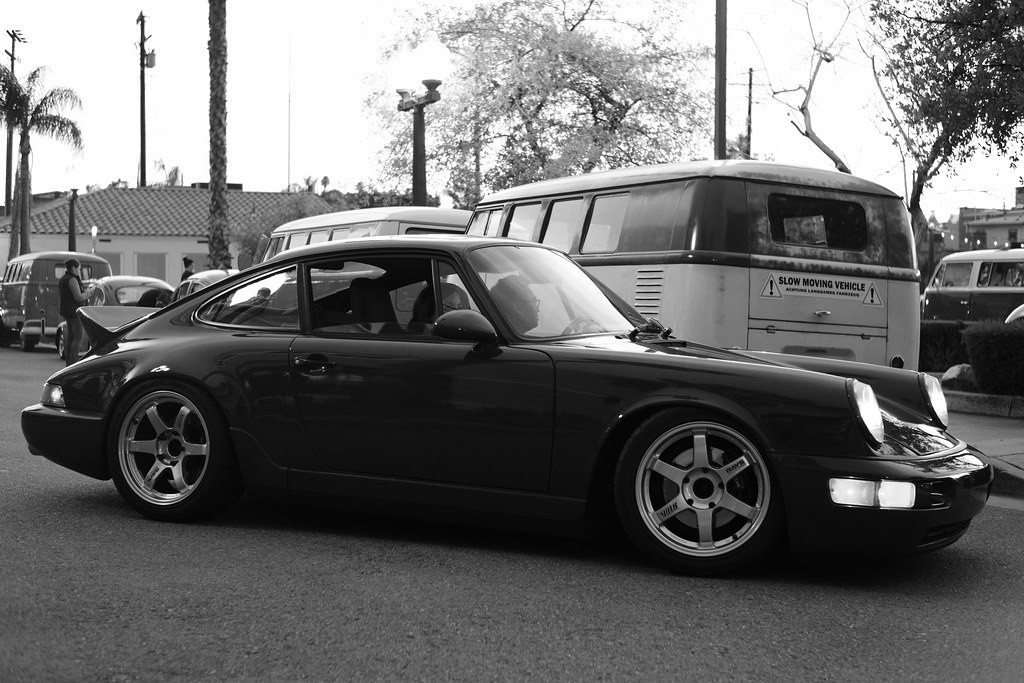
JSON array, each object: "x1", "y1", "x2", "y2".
[
  {"x1": 54, "y1": 275, "x2": 176, "y2": 359},
  {"x1": 167, "y1": 268, "x2": 241, "y2": 307},
  {"x1": 20, "y1": 233, "x2": 997, "y2": 580}
]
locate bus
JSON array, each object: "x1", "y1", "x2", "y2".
[{"x1": 465, "y1": 158, "x2": 922, "y2": 375}]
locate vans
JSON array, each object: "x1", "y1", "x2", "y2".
[
  {"x1": 263, "y1": 205, "x2": 474, "y2": 270},
  {"x1": 922, "y1": 245, "x2": 1023, "y2": 331},
  {"x1": 0, "y1": 251, "x2": 114, "y2": 352}
]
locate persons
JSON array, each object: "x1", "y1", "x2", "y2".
[
  {"x1": 490, "y1": 275, "x2": 540, "y2": 334},
  {"x1": 406, "y1": 283, "x2": 471, "y2": 334},
  {"x1": 794, "y1": 216, "x2": 817, "y2": 244},
  {"x1": 58, "y1": 258, "x2": 96, "y2": 367},
  {"x1": 181, "y1": 257, "x2": 194, "y2": 282}
]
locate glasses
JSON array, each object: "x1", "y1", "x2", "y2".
[{"x1": 515, "y1": 297, "x2": 540, "y2": 308}]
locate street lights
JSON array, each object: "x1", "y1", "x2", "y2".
[
  {"x1": 136, "y1": 10, "x2": 156, "y2": 185},
  {"x1": 395, "y1": 77, "x2": 442, "y2": 206}
]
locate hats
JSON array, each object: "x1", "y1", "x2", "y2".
[{"x1": 182, "y1": 257, "x2": 193, "y2": 267}]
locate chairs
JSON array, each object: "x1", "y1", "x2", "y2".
[{"x1": 350, "y1": 278, "x2": 404, "y2": 333}]
